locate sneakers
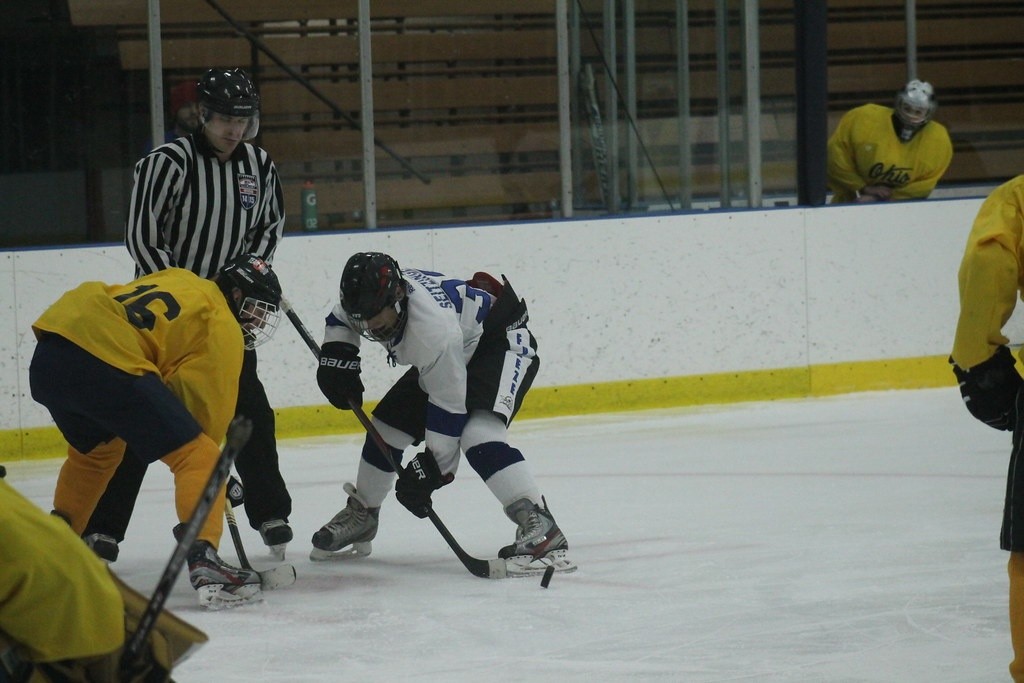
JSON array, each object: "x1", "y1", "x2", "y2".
[
  {"x1": 497, "y1": 494, "x2": 578, "y2": 575},
  {"x1": 172, "y1": 522, "x2": 262, "y2": 612},
  {"x1": 308, "y1": 482, "x2": 381, "y2": 562},
  {"x1": 83, "y1": 533, "x2": 120, "y2": 568},
  {"x1": 258, "y1": 518, "x2": 293, "y2": 560}
]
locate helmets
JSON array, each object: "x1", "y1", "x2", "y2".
[
  {"x1": 216, "y1": 253, "x2": 283, "y2": 352},
  {"x1": 195, "y1": 64, "x2": 261, "y2": 141},
  {"x1": 341, "y1": 250, "x2": 410, "y2": 346},
  {"x1": 892, "y1": 79, "x2": 939, "y2": 142}
]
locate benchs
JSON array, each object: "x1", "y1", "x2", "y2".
[{"x1": 67, "y1": 0, "x2": 1024, "y2": 233}]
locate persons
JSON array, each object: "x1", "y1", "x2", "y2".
[
  {"x1": 824, "y1": 80, "x2": 953, "y2": 206},
  {"x1": 947, "y1": 174, "x2": 1024, "y2": 682},
  {"x1": 307, "y1": 252, "x2": 578, "y2": 575},
  {"x1": 0, "y1": 468, "x2": 210, "y2": 683},
  {"x1": 124, "y1": 68, "x2": 293, "y2": 561},
  {"x1": 27, "y1": 254, "x2": 282, "y2": 610}
]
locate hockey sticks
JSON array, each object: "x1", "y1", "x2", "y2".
[
  {"x1": 275, "y1": 293, "x2": 508, "y2": 578},
  {"x1": 540, "y1": 566, "x2": 555, "y2": 588},
  {"x1": 581, "y1": 63, "x2": 611, "y2": 210},
  {"x1": 120, "y1": 415, "x2": 251, "y2": 668},
  {"x1": 223, "y1": 497, "x2": 296, "y2": 590}
]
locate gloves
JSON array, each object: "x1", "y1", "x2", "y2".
[
  {"x1": 226, "y1": 475, "x2": 247, "y2": 507},
  {"x1": 947, "y1": 345, "x2": 1024, "y2": 431},
  {"x1": 316, "y1": 341, "x2": 365, "y2": 411},
  {"x1": 393, "y1": 446, "x2": 455, "y2": 518}
]
